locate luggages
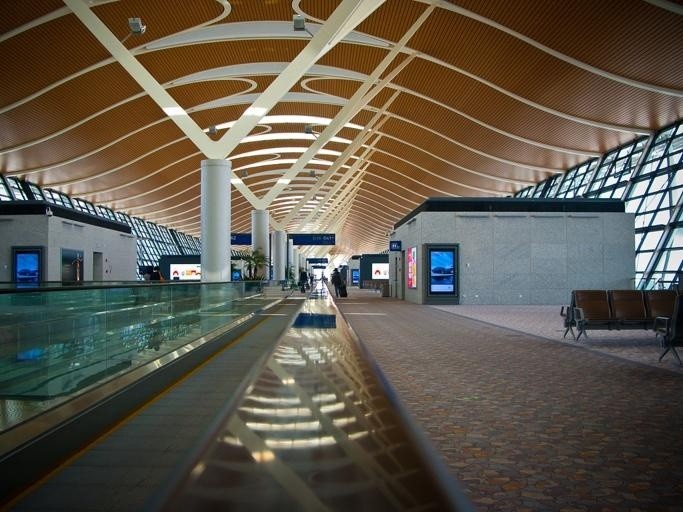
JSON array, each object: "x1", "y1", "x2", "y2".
[{"x1": 339, "y1": 287, "x2": 347, "y2": 297}]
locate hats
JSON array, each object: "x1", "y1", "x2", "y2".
[{"x1": 333, "y1": 268, "x2": 338, "y2": 271}]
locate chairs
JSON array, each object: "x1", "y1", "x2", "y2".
[
  {"x1": 560, "y1": 289, "x2": 683, "y2": 367},
  {"x1": 362, "y1": 279, "x2": 389, "y2": 293}
]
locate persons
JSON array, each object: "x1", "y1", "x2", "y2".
[
  {"x1": 299, "y1": 267, "x2": 316, "y2": 293},
  {"x1": 340, "y1": 265, "x2": 347, "y2": 297},
  {"x1": 142, "y1": 266, "x2": 162, "y2": 283},
  {"x1": 147, "y1": 329, "x2": 166, "y2": 351},
  {"x1": 331, "y1": 268, "x2": 342, "y2": 297}
]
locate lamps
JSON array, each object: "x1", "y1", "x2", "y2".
[
  {"x1": 293, "y1": 14, "x2": 313, "y2": 37},
  {"x1": 305, "y1": 125, "x2": 318, "y2": 139},
  {"x1": 240, "y1": 169, "x2": 249, "y2": 179},
  {"x1": 310, "y1": 168, "x2": 319, "y2": 181},
  {"x1": 206, "y1": 125, "x2": 216, "y2": 135},
  {"x1": 120, "y1": 17, "x2": 146, "y2": 44}
]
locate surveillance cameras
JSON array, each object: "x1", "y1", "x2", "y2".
[
  {"x1": 385, "y1": 230, "x2": 389, "y2": 237},
  {"x1": 47, "y1": 211, "x2": 53, "y2": 217}
]
